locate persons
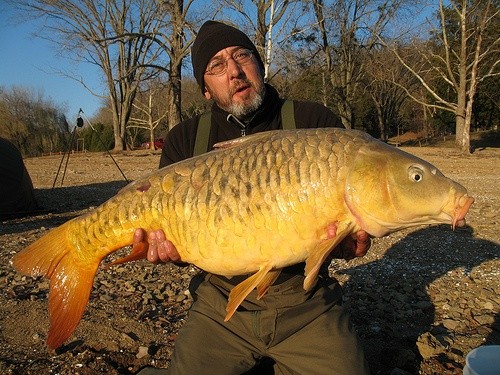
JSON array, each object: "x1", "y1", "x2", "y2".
[{"x1": 132, "y1": 20, "x2": 372, "y2": 375}]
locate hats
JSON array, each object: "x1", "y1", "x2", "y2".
[{"x1": 192, "y1": 19, "x2": 266, "y2": 96}]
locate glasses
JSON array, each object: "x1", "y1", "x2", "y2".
[{"x1": 204, "y1": 49, "x2": 256, "y2": 75}]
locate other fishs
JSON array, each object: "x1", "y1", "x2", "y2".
[{"x1": 12, "y1": 128, "x2": 476, "y2": 354}]
